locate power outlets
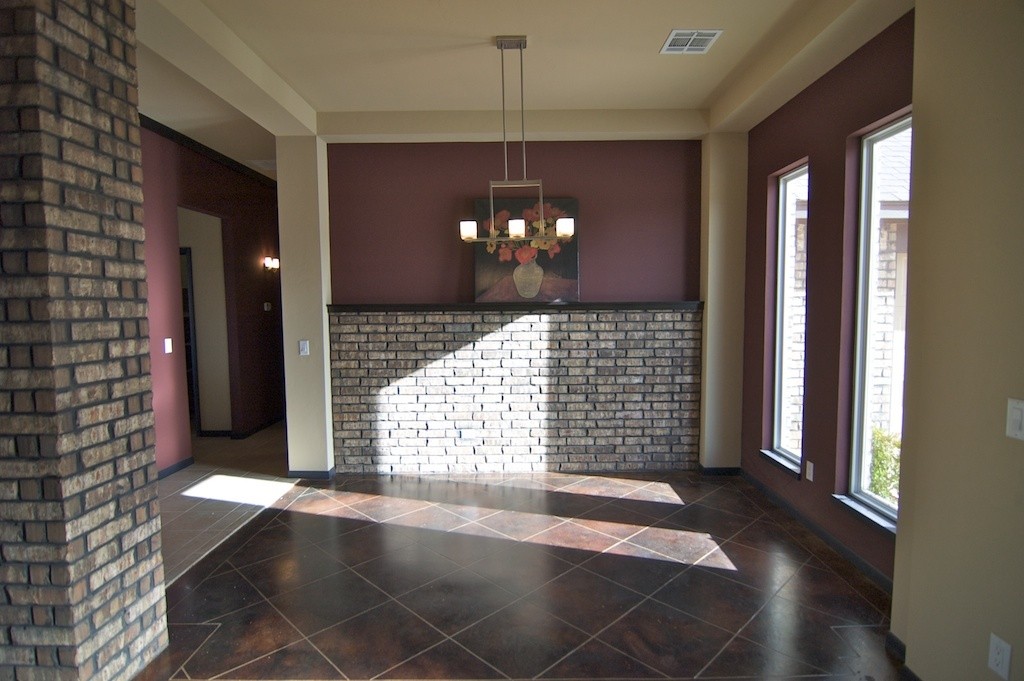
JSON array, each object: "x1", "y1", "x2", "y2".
[{"x1": 988, "y1": 633, "x2": 1012, "y2": 681}]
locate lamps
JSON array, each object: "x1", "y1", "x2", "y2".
[
  {"x1": 460, "y1": 36, "x2": 574, "y2": 243},
  {"x1": 264, "y1": 256, "x2": 279, "y2": 273}
]
